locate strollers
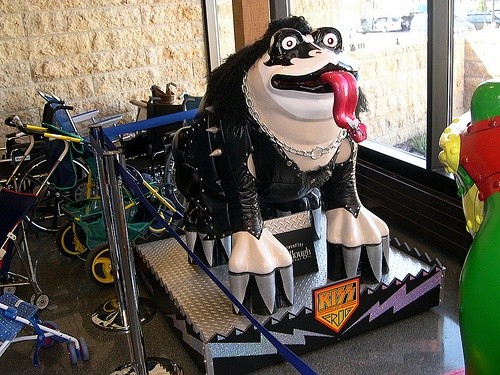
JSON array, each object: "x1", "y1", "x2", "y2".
[
  {"x1": 0, "y1": 90, "x2": 203, "y2": 311},
  {"x1": 0, "y1": 292, "x2": 90, "y2": 366}
]
